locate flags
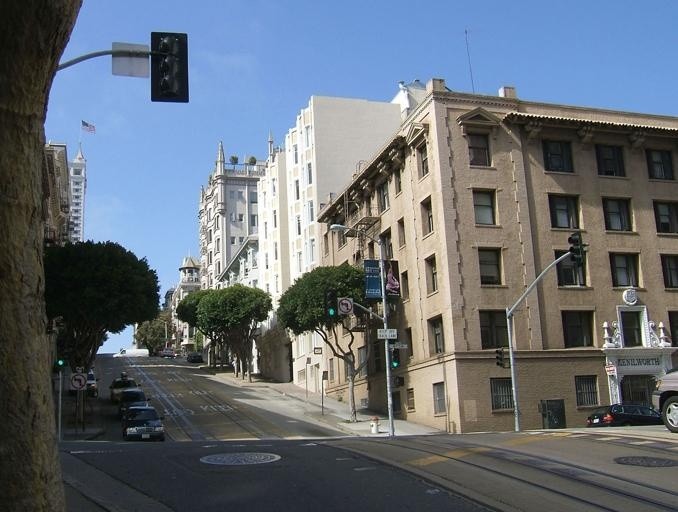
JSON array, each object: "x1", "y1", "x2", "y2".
[{"x1": 82, "y1": 120, "x2": 96, "y2": 136}]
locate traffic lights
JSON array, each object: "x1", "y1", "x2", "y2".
[
  {"x1": 494, "y1": 348, "x2": 505, "y2": 368},
  {"x1": 324, "y1": 288, "x2": 338, "y2": 321},
  {"x1": 565, "y1": 230, "x2": 584, "y2": 266},
  {"x1": 56, "y1": 356, "x2": 66, "y2": 370},
  {"x1": 392, "y1": 348, "x2": 401, "y2": 368}
]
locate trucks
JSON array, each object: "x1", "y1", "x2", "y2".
[{"x1": 113, "y1": 348, "x2": 149, "y2": 358}]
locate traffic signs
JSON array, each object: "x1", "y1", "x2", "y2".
[
  {"x1": 390, "y1": 342, "x2": 408, "y2": 350},
  {"x1": 377, "y1": 329, "x2": 398, "y2": 340}
]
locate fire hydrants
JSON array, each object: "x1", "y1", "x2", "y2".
[{"x1": 368, "y1": 415, "x2": 380, "y2": 434}]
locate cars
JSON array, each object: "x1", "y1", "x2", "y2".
[
  {"x1": 107, "y1": 370, "x2": 166, "y2": 442},
  {"x1": 85, "y1": 365, "x2": 100, "y2": 398},
  {"x1": 649, "y1": 368, "x2": 677, "y2": 434},
  {"x1": 186, "y1": 352, "x2": 204, "y2": 363},
  {"x1": 161, "y1": 348, "x2": 176, "y2": 358},
  {"x1": 173, "y1": 353, "x2": 181, "y2": 359}
]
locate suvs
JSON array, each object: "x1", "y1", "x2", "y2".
[{"x1": 583, "y1": 404, "x2": 665, "y2": 428}]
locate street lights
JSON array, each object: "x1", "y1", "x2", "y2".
[{"x1": 328, "y1": 223, "x2": 395, "y2": 440}]
